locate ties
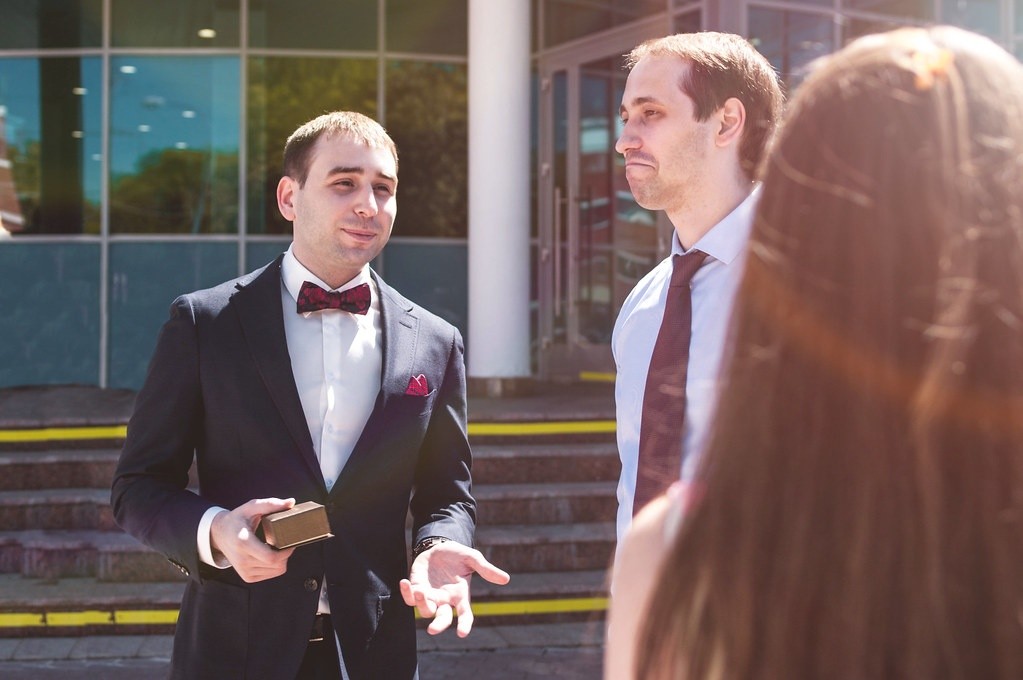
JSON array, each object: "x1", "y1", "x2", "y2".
[{"x1": 632, "y1": 249, "x2": 707, "y2": 522}]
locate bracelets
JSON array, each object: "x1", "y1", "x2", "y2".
[{"x1": 411, "y1": 536, "x2": 450, "y2": 563}]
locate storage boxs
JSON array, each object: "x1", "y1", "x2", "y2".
[{"x1": 262, "y1": 501, "x2": 335, "y2": 549}]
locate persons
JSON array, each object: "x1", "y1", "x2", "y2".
[
  {"x1": 109, "y1": 112, "x2": 510, "y2": 680},
  {"x1": 612, "y1": 31, "x2": 785, "y2": 552},
  {"x1": 602, "y1": 23, "x2": 1023, "y2": 680}
]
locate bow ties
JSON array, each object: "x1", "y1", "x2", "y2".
[{"x1": 295, "y1": 280, "x2": 372, "y2": 315}]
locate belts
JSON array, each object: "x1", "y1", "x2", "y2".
[{"x1": 306, "y1": 612, "x2": 335, "y2": 645}]
